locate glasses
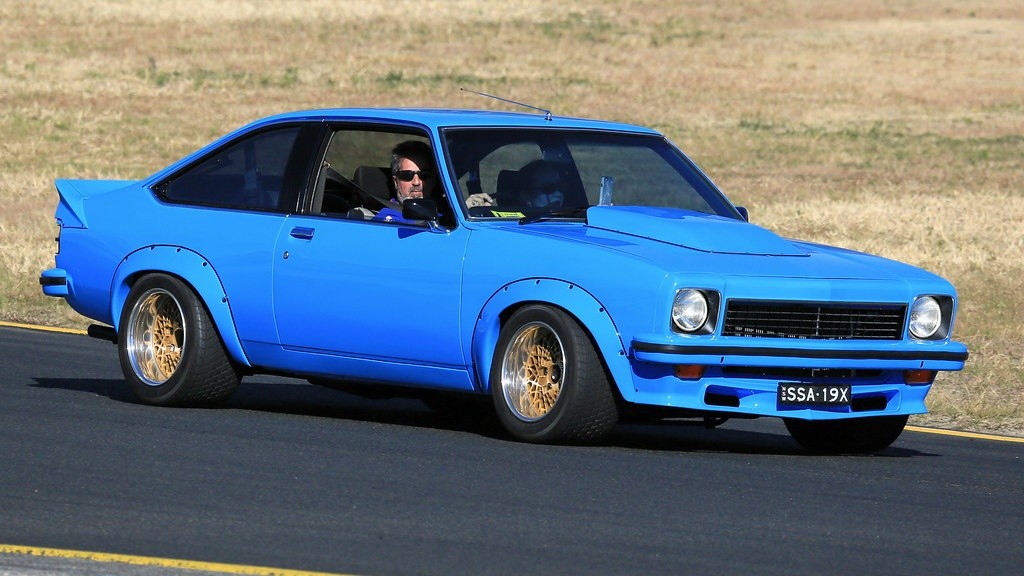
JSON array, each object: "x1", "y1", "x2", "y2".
[
  {"x1": 394, "y1": 169, "x2": 434, "y2": 181},
  {"x1": 541, "y1": 181, "x2": 569, "y2": 194}
]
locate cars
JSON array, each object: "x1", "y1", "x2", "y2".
[{"x1": 39, "y1": 107, "x2": 969, "y2": 456}]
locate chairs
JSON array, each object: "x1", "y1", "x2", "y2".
[
  {"x1": 496, "y1": 170, "x2": 523, "y2": 205},
  {"x1": 354, "y1": 165, "x2": 396, "y2": 198}
]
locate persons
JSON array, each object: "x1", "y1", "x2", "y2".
[
  {"x1": 514, "y1": 159, "x2": 567, "y2": 216},
  {"x1": 371, "y1": 141, "x2": 493, "y2": 225}
]
